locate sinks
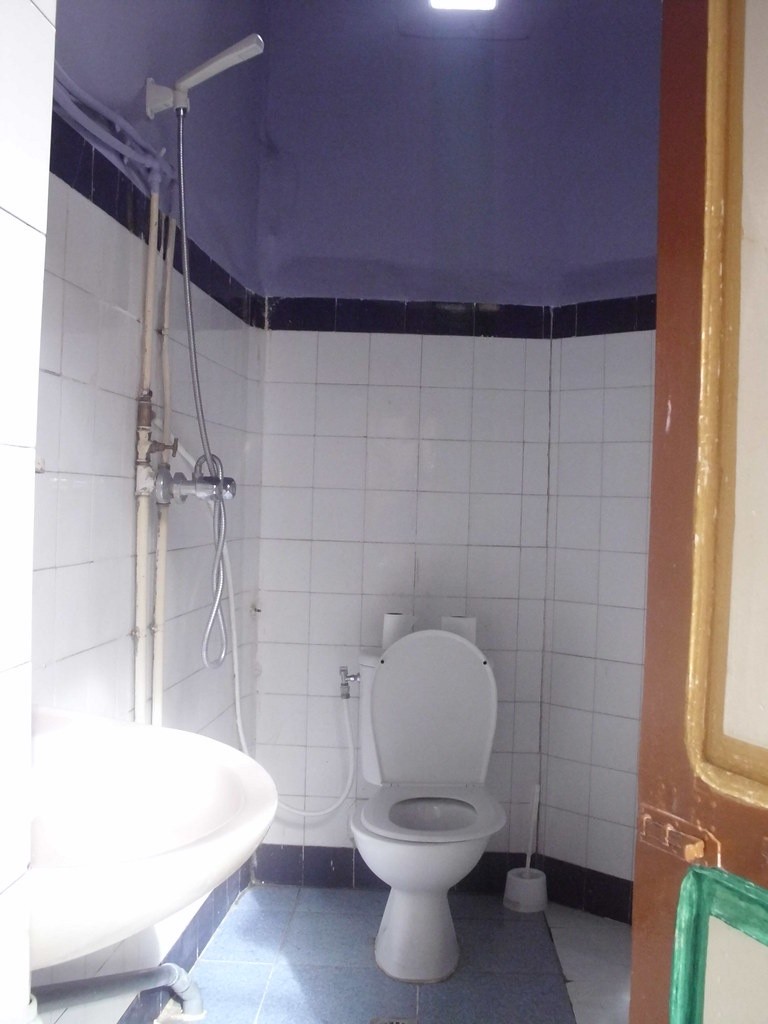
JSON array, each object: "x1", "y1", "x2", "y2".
[{"x1": 29, "y1": 709, "x2": 280, "y2": 986}]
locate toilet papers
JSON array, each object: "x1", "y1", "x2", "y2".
[{"x1": 379, "y1": 611, "x2": 480, "y2": 668}]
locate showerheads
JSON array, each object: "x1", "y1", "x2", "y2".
[{"x1": 173, "y1": 32, "x2": 265, "y2": 95}]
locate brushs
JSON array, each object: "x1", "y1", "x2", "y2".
[{"x1": 504, "y1": 782, "x2": 551, "y2": 915}]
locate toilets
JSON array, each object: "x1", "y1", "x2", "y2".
[{"x1": 349, "y1": 628, "x2": 509, "y2": 983}]
